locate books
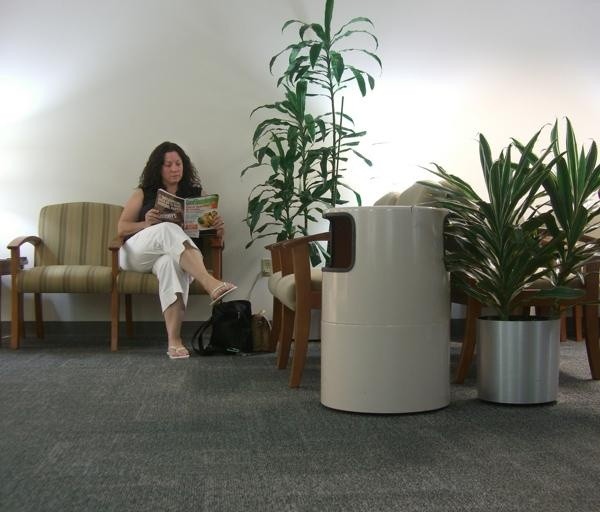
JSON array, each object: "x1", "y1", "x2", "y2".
[{"x1": 154, "y1": 189, "x2": 219, "y2": 239}]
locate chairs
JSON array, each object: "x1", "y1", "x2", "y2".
[
  {"x1": 7, "y1": 202, "x2": 224, "y2": 352},
  {"x1": 263, "y1": 178, "x2": 600, "y2": 389}
]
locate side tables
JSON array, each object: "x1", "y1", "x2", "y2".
[{"x1": 0, "y1": 256, "x2": 27, "y2": 339}]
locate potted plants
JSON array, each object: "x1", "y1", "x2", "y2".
[
  {"x1": 416, "y1": 115, "x2": 600, "y2": 407},
  {"x1": 239, "y1": 0, "x2": 382, "y2": 343}
]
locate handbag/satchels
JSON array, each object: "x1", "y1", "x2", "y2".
[{"x1": 251, "y1": 312, "x2": 273, "y2": 354}]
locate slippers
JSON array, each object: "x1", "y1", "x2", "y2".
[
  {"x1": 166, "y1": 346, "x2": 191, "y2": 359},
  {"x1": 209, "y1": 282, "x2": 239, "y2": 307}
]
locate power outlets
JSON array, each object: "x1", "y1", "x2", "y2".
[{"x1": 262, "y1": 258, "x2": 273, "y2": 277}]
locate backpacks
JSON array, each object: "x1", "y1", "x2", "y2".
[{"x1": 190, "y1": 299, "x2": 253, "y2": 356}]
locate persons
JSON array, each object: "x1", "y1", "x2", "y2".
[{"x1": 118, "y1": 142, "x2": 237, "y2": 360}]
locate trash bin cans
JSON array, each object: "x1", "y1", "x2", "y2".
[{"x1": 321, "y1": 205, "x2": 452, "y2": 414}]
